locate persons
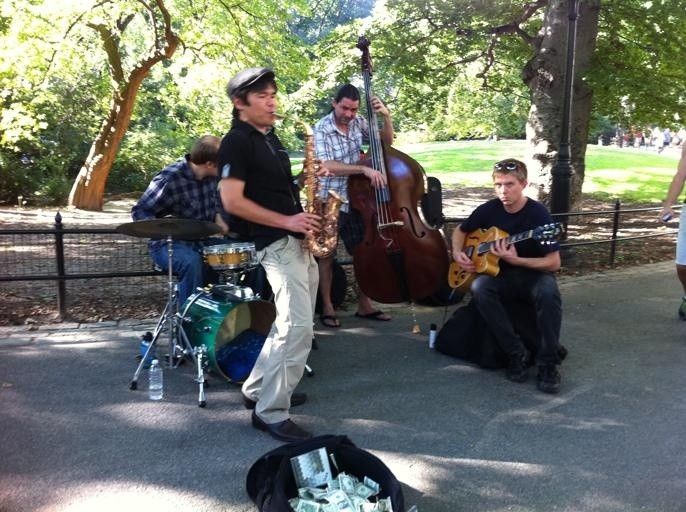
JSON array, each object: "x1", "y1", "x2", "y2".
[
  {"x1": 311, "y1": 84, "x2": 393, "y2": 327},
  {"x1": 623, "y1": 128, "x2": 673, "y2": 148},
  {"x1": 659, "y1": 140, "x2": 686, "y2": 318},
  {"x1": 130, "y1": 135, "x2": 230, "y2": 368},
  {"x1": 451, "y1": 158, "x2": 567, "y2": 393},
  {"x1": 219, "y1": 67, "x2": 334, "y2": 443}
]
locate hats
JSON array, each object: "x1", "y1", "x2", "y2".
[{"x1": 225, "y1": 67, "x2": 276, "y2": 98}]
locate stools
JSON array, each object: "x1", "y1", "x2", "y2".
[{"x1": 148, "y1": 260, "x2": 181, "y2": 363}]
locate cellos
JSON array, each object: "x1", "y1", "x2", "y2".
[{"x1": 348, "y1": 35, "x2": 453, "y2": 304}]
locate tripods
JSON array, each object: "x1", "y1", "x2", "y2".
[{"x1": 129, "y1": 251, "x2": 210, "y2": 389}]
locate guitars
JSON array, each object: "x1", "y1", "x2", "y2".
[{"x1": 448, "y1": 222, "x2": 566, "y2": 294}]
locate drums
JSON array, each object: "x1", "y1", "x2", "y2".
[
  {"x1": 176, "y1": 283, "x2": 275, "y2": 387},
  {"x1": 204, "y1": 243, "x2": 255, "y2": 270}
]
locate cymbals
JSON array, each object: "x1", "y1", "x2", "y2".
[{"x1": 113, "y1": 218, "x2": 223, "y2": 241}]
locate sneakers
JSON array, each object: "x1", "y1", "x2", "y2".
[
  {"x1": 536, "y1": 355, "x2": 562, "y2": 394},
  {"x1": 507, "y1": 345, "x2": 530, "y2": 383}
]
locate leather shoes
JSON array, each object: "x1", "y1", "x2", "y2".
[
  {"x1": 243, "y1": 391, "x2": 309, "y2": 410},
  {"x1": 250, "y1": 407, "x2": 314, "y2": 444}
]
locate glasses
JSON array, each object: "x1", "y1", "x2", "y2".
[{"x1": 494, "y1": 162, "x2": 518, "y2": 171}]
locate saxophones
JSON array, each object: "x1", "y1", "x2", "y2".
[{"x1": 271, "y1": 111, "x2": 350, "y2": 261}]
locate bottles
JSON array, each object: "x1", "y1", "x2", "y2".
[
  {"x1": 149, "y1": 360, "x2": 166, "y2": 400},
  {"x1": 428, "y1": 322, "x2": 437, "y2": 349},
  {"x1": 140, "y1": 332, "x2": 153, "y2": 366}
]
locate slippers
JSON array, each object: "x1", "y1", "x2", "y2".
[
  {"x1": 354, "y1": 308, "x2": 394, "y2": 323},
  {"x1": 319, "y1": 312, "x2": 342, "y2": 329}
]
